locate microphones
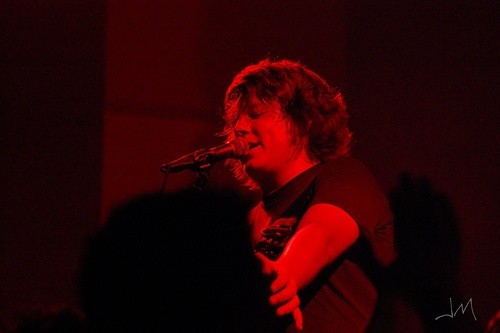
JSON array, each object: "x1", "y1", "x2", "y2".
[{"x1": 160, "y1": 136, "x2": 251, "y2": 175}]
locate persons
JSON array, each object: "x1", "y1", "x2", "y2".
[{"x1": 214, "y1": 59, "x2": 395, "y2": 332}]
